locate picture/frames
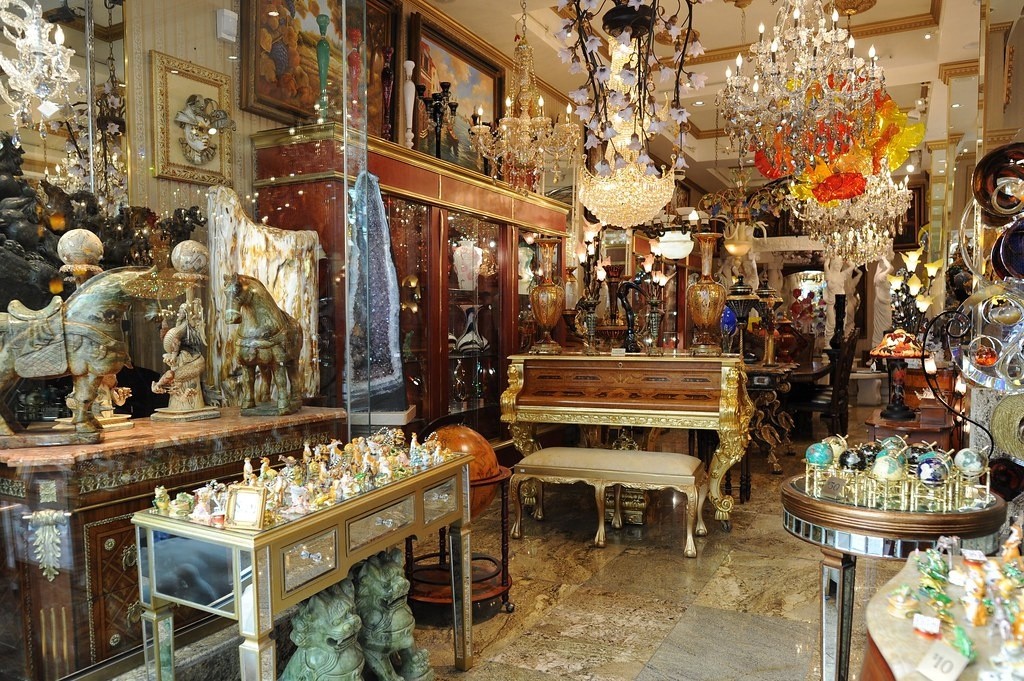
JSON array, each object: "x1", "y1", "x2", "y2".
[
  {"x1": 240, "y1": 0, "x2": 405, "y2": 144},
  {"x1": 148, "y1": 49, "x2": 234, "y2": 190},
  {"x1": 407, "y1": 10, "x2": 507, "y2": 181},
  {"x1": 654, "y1": 164, "x2": 692, "y2": 225},
  {"x1": 223, "y1": 484, "x2": 268, "y2": 531}
]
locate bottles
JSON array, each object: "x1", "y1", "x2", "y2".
[
  {"x1": 456, "y1": 305, "x2": 490, "y2": 354},
  {"x1": 528, "y1": 238, "x2": 565, "y2": 355},
  {"x1": 454, "y1": 240, "x2": 482, "y2": 290},
  {"x1": 686, "y1": 232, "x2": 727, "y2": 356}
]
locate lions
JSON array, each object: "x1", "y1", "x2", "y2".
[{"x1": 276, "y1": 548, "x2": 435, "y2": 681}]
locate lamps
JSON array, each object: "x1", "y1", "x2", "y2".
[
  {"x1": 714, "y1": 0, "x2": 886, "y2": 178},
  {"x1": 47, "y1": 0, "x2": 128, "y2": 217},
  {"x1": 869, "y1": 328, "x2": 931, "y2": 421},
  {"x1": 697, "y1": 168, "x2": 785, "y2": 257},
  {"x1": 750, "y1": 0, "x2": 925, "y2": 210},
  {"x1": 578, "y1": 38, "x2": 676, "y2": 229},
  {"x1": 528, "y1": 238, "x2": 566, "y2": 355},
  {"x1": 797, "y1": 170, "x2": 913, "y2": 268},
  {"x1": 886, "y1": 242, "x2": 944, "y2": 336},
  {"x1": 641, "y1": 253, "x2": 670, "y2": 358},
  {"x1": 789, "y1": 289, "x2": 827, "y2": 365},
  {"x1": 0, "y1": 0, "x2": 86, "y2": 148},
  {"x1": 658, "y1": 231, "x2": 694, "y2": 349},
  {"x1": 907, "y1": 103, "x2": 928, "y2": 121},
  {"x1": 469, "y1": 0, "x2": 582, "y2": 198},
  {"x1": 636, "y1": 132, "x2": 713, "y2": 239},
  {"x1": 575, "y1": 231, "x2": 607, "y2": 356}
]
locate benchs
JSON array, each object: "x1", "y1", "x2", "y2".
[{"x1": 509, "y1": 446, "x2": 713, "y2": 558}]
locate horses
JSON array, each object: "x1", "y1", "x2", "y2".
[
  {"x1": 222, "y1": 272, "x2": 305, "y2": 414},
  {"x1": 0, "y1": 265, "x2": 164, "y2": 436}
]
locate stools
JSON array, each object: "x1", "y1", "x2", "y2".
[{"x1": 849, "y1": 373, "x2": 888, "y2": 407}]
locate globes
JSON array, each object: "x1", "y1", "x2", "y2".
[
  {"x1": 801, "y1": 431, "x2": 992, "y2": 513},
  {"x1": 398, "y1": 411, "x2": 517, "y2": 619}
]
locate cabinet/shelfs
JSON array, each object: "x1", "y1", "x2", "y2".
[{"x1": 248, "y1": 120, "x2": 573, "y2": 474}]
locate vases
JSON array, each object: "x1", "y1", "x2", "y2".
[
  {"x1": 686, "y1": 233, "x2": 726, "y2": 357},
  {"x1": 453, "y1": 240, "x2": 482, "y2": 291},
  {"x1": 457, "y1": 303, "x2": 490, "y2": 354}
]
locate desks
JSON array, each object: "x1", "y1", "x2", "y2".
[
  {"x1": 500, "y1": 351, "x2": 757, "y2": 533},
  {"x1": 778, "y1": 362, "x2": 830, "y2": 440},
  {"x1": 780, "y1": 473, "x2": 1008, "y2": 681},
  {"x1": 745, "y1": 362, "x2": 801, "y2": 475},
  {"x1": 0, "y1": 407, "x2": 347, "y2": 681},
  {"x1": 129, "y1": 451, "x2": 476, "y2": 681},
  {"x1": 864, "y1": 406, "x2": 955, "y2": 455}
]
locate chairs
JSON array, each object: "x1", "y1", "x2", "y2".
[{"x1": 789, "y1": 328, "x2": 862, "y2": 438}]
